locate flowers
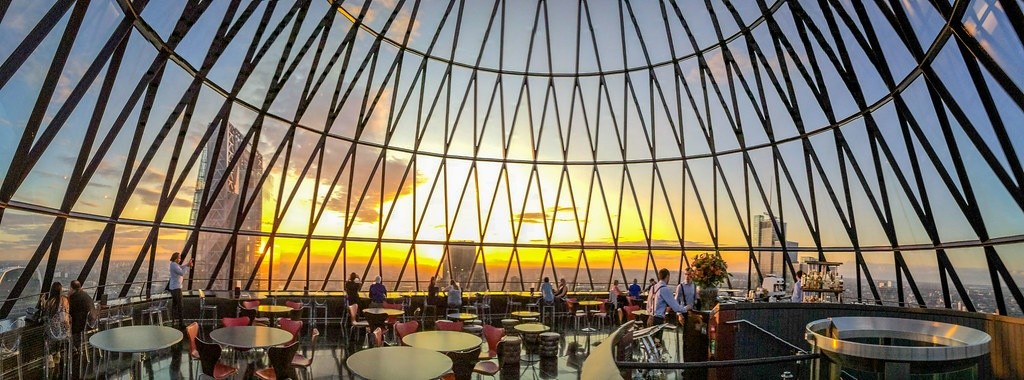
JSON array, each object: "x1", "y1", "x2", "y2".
[{"x1": 685, "y1": 253, "x2": 734, "y2": 290}]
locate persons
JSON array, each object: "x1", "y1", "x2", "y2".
[
  {"x1": 647, "y1": 268, "x2": 699, "y2": 344},
  {"x1": 644, "y1": 278, "x2": 657, "y2": 293},
  {"x1": 369, "y1": 276, "x2": 387, "y2": 304},
  {"x1": 443, "y1": 280, "x2": 462, "y2": 312},
  {"x1": 791, "y1": 271, "x2": 802, "y2": 302},
  {"x1": 345, "y1": 272, "x2": 362, "y2": 307},
  {"x1": 45, "y1": 279, "x2": 95, "y2": 367},
  {"x1": 629, "y1": 279, "x2": 642, "y2": 299},
  {"x1": 540, "y1": 277, "x2": 554, "y2": 306},
  {"x1": 613, "y1": 280, "x2": 623, "y2": 302},
  {"x1": 429, "y1": 277, "x2": 442, "y2": 309},
  {"x1": 554, "y1": 279, "x2": 568, "y2": 301},
  {"x1": 170, "y1": 253, "x2": 193, "y2": 322}
]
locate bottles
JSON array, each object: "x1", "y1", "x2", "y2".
[
  {"x1": 693, "y1": 291, "x2": 702, "y2": 311},
  {"x1": 801, "y1": 269, "x2": 844, "y2": 291}
]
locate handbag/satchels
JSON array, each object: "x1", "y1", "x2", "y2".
[
  {"x1": 25, "y1": 307, "x2": 40, "y2": 322},
  {"x1": 165, "y1": 280, "x2": 171, "y2": 291}
]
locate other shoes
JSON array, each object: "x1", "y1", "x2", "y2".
[
  {"x1": 48, "y1": 355, "x2": 54, "y2": 369},
  {"x1": 53, "y1": 351, "x2": 61, "y2": 363}
]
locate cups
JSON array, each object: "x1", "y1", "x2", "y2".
[
  {"x1": 234, "y1": 287, "x2": 240, "y2": 295},
  {"x1": 749, "y1": 287, "x2": 769, "y2": 299},
  {"x1": 44, "y1": 293, "x2": 49, "y2": 300},
  {"x1": 530, "y1": 288, "x2": 534, "y2": 292}
]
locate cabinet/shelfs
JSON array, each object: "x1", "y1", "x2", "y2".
[{"x1": 802, "y1": 259, "x2": 845, "y2": 305}]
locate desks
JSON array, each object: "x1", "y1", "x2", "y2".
[
  {"x1": 578, "y1": 301, "x2": 603, "y2": 331},
  {"x1": 401, "y1": 288, "x2": 569, "y2": 324},
  {"x1": 447, "y1": 313, "x2": 478, "y2": 324},
  {"x1": 89, "y1": 325, "x2": 183, "y2": 380},
  {"x1": 362, "y1": 308, "x2": 405, "y2": 316},
  {"x1": 514, "y1": 324, "x2": 550, "y2": 362},
  {"x1": 346, "y1": 346, "x2": 453, "y2": 380},
  {"x1": 402, "y1": 330, "x2": 482, "y2": 356},
  {"x1": 252, "y1": 305, "x2": 294, "y2": 327},
  {"x1": 210, "y1": 325, "x2": 293, "y2": 371},
  {"x1": 511, "y1": 311, "x2": 541, "y2": 317}
]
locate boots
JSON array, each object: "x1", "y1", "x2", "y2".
[
  {"x1": 171, "y1": 308, "x2": 177, "y2": 327},
  {"x1": 178, "y1": 308, "x2": 188, "y2": 329}
]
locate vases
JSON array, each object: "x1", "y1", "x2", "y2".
[{"x1": 696, "y1": 284, "x2": 719, "y2": 310}]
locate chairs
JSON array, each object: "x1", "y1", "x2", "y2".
[
  {"x1": 348, "y1": 302, "x2": 506, "y2": 380},
  {"x1": 186, "y1": 300, "x2": 319, "y2": 379},
  {"x1": 566, "y1": 292, "x2": 648, "y2": 330}
]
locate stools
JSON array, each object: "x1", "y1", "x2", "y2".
[
  {"x1": 463, "y1": 318, "x2": 561, "y2": 367},
  {"x1": 198, "y1": 289, "x2": 218, "y2": 326},
  {"x1": 0, "y1": 290, "x2": 170, "y2": 380},
  {"x1": 236, "y1": 289, "x2": 353, "y2": 327}
]
